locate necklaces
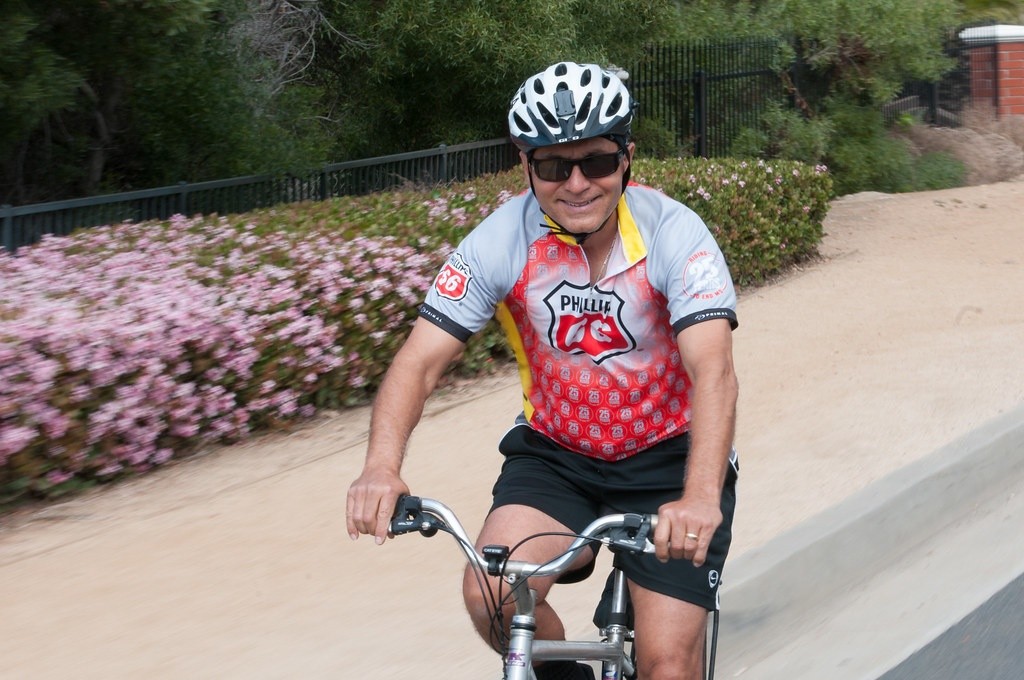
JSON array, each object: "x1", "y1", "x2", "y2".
[{"x1": 594, "y1": 231, "x2": 618, "y2": 290}]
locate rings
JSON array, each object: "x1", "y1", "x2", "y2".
[{"x1": 686, "y1": 533, "x2": 699, "y2": 542}]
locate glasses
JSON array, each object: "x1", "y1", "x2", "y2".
[{"x1": 529, "y1": 145, "x2": 629, "y2": 181}]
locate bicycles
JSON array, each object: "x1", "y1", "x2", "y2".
[{"x1": 388, "y1": 495, "x2": 718, "y2": 680}]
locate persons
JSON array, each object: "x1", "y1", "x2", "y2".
[{"x1": 344, "y1": 60, "x2": 740, "y2": 679}]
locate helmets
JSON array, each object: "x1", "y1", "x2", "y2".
[{"x1": 507, "y1": 61, "x2": 635, "y2": 154}]
